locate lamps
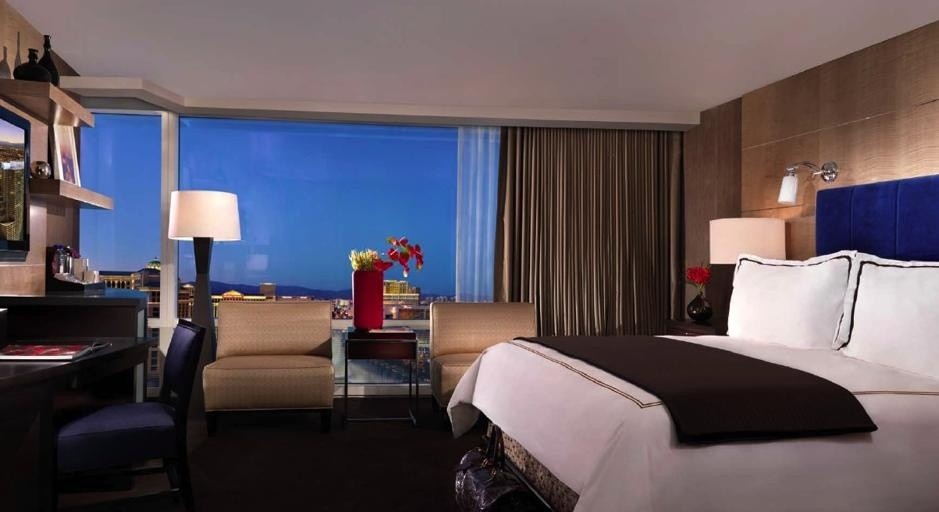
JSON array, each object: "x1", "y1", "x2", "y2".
[
  {"x1": 778, "y1": 159, "x2": 840, "y2": 207},
  {"x1": 166, "y1": 190, "x2": 243, "y2": 418},
  {"x1": 708, "y1": 217, "x2": 786, "y2": 265}
]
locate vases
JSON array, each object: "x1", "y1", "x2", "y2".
[
  {"x1": 351, "y1": 271, "x2": 384, "y2": 329},
  {"x1": 32, "y1": 34, "x2": 61, "y2": 86},
  {"x1": 12, "y1": 48, "x2": 39, "y2": 81},
  {"x1": 687, "y1": 291, "x2": 713, "y2": 325}
]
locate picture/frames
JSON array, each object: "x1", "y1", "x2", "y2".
[{"x1": 52, "y1": 123, "x2": 82, "y2": 186}]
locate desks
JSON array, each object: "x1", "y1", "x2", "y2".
[
  {"x1": 0, "y1": 337, "x2": 151, "y2": 512},
  {"x1": 343, "y1": 325, "x2": 421, "y2": 431}
]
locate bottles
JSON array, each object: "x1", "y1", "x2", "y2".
[
  {"x1": 37, "y1": 35, "x2": 60, "y2": 87},
  {"x1": 13, "y1": 48, "x2": 51, "y2": 82}
]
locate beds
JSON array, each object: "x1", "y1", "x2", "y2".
[{"x1": 446, "y1": 173, "x2": 939, "y2": 512}]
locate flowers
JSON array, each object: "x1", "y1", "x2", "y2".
[
  {"x1": 348, "y1": 235, "x2": 424, "y2": 278},
  {"x1": 680, "y1": 261, "x2": 713, "y2": 294}
]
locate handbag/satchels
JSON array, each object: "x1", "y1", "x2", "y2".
[{"x1": 454, "y1": 424, "x2": 531, "y2": 510}]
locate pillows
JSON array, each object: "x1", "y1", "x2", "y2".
[
  {"x1": 830, "y1": 252, "x2": 939, "y2": 380},
  {"x1": 726, "y1": 249, "x2": 856, "y2": 350}
]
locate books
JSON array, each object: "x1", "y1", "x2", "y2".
[{"x1": 0, "y1": 343, "x2": 92, "y2": 361}]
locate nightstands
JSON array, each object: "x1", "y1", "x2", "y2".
[{"x1": 666, "y1": 316, "x2": 727, "y2": 336}]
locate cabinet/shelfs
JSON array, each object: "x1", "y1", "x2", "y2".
[
  {"x1": 0, "y1": 288, "x2": 149, "y2": 493},
  {"x1": 0, "y1": 78, "x2": 115, "y2": 211}
]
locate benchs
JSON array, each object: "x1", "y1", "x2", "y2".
[
  {"x1": 429, "y1": 300, "x2": 538, "y2": 432},
  {"x1": 201, "y1": 300, "x2": 334, "y2": 436}
]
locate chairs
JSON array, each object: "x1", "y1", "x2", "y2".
[{"x1": 39, "y1": 318, "x2": 208, "y2": 512}]
